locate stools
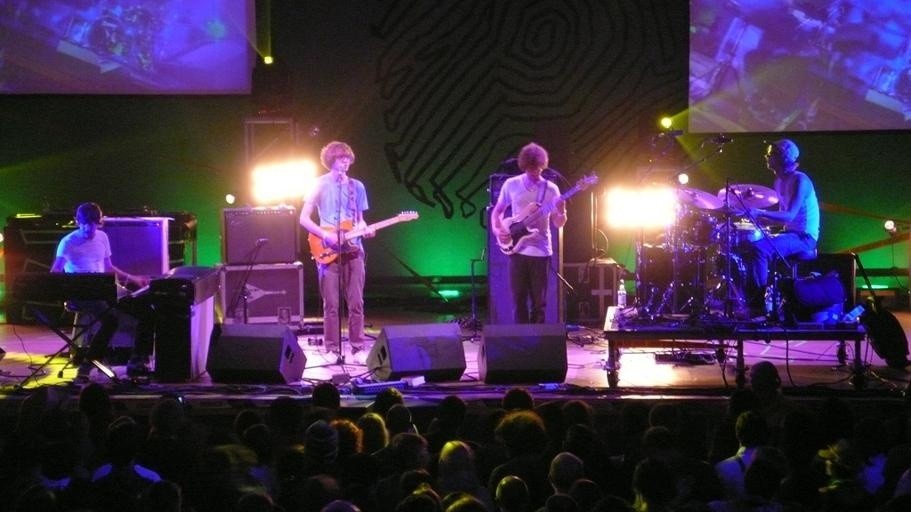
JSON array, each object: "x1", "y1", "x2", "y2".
[
  {"x1": 782, "y1": 249, "x2": 817, "y2": 328},
  {"x1": 57, "y1": 299, "x2": 118, "y2": 380}
]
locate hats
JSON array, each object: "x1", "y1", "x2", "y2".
[{"x1": 763, "y1": 139, "x2": 800, "y2": 162}]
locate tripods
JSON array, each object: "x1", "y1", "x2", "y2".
[{"x1": 304, "y1": 181, "x2": 367, "y2": 375}]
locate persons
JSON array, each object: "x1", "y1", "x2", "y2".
[
  {"x1": 490, "y1": 142, "x2": 567, "y2": 324},
  {"x1": 1, "y1": 356, "x2": 911, "y2": 512},
  {"x1": 299, "y1": 141, "x2": 376, "y2": 364},
  {"x1": 746, "y1": 138, "x2": 821, "y2": 296},
  {"x1": 50, "y1": 201, "x2": 157, "y2": 386}
]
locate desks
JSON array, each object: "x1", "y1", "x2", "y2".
[{"x1": 603, "y1": 306, "x2": 865, "y2": 389}]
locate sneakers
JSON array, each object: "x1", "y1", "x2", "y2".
[{"x1": 353, "y1": 352, "x2": 366, "y2": 365}]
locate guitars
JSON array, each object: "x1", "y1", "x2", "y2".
[
  {"x1": 495, "y1": 174, "x2": 599, "y2": 256},
  {"x1": 854, "y1": 253, "x2": 910, "y2": 371},
  {"x1": 307, "y1": 211, "x2": 419, "y2": 265}
]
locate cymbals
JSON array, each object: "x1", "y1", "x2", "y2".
[
  {"x1": 716, "y1": 220, "x2": 765, "y2": 245},
  {"x1": 679, "y1": 187, "x2": 725, "y2": 211},
  {"x1": 718, "y1": 183, "x2": 779, "y2": 210}
]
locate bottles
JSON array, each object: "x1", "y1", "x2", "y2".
[
  {"x1": 617, "y1": 279, "x2": 627, "y2": 309},
  {"x1": 842, "y1": 305, "x2": 865, "y2": 321}
]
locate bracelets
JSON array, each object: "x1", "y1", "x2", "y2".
[
  {"x1": 557, "y1": 210, "x2": 567, "y2": 217},
  {"x1": 125, "y1": 273, "x2": 130, "y2": 282},
  {"x1": 762, "y1": 209, "x2": 767, "y2": 217},
  {"x1": 322, "y1": 231, "x2": 328, "y2": 239}
]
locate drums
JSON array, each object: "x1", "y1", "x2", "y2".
[
  {"x1": 674, "y1": 209, "x2": 716, "y2": 244},
  {"x1": 636, "y1": 242, "x2": 673, "y2": 283},
  {"x1": 677, "y1": 247, "x2": 705, "y2": 280}
]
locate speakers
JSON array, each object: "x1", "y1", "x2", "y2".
[
  {"x1": 486, "y1": 206, "x2": 566, "y2": 324},
  {"x1": 365, "y1": 323, "x2": 466, "y2": 382},
  {"x1": 478, "y1": 323, "x2": 568, "y2": 384},
  {"x1": 205, "y1": 322, "x2": 307, "y2": 383},
  {"x1": 220, "y1": 204, "x2": 302, "y2": 265}
]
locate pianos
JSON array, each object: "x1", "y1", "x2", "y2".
[
  {"x1": 11, "y1": 271, "x2": 119, "y2": 303},
  {"x1": 117, "y1": 266, "x2": 220, "y2": 383}
]
